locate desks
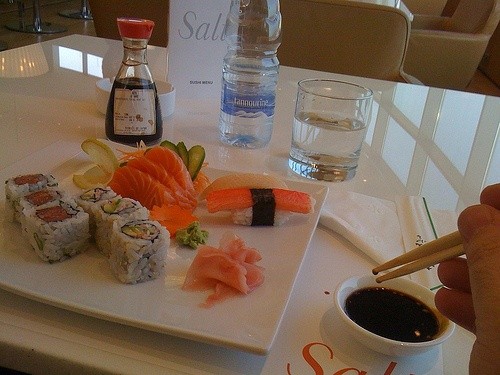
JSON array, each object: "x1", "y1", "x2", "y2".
[{"x1": 0, "y1": 33, "x2": 500, "y2": 375}]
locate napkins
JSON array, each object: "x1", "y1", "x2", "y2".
[{"x1": 318, "y1": 188, "x2": 439, "y2": 280}]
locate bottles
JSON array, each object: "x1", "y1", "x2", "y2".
[
  {"x1": 104, "y1": 18, "x2": 162, "y2": 147},
  {"x1": 219, "y1": 0, "x2": 282, "y2": 148}
]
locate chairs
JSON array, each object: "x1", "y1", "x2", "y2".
[{"x1": 87, "y1": 0, "x2": 500, "y2": 91}]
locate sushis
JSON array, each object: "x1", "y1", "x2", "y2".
[
  {"x1": 197, "y1": 175, "x2": 317, "y2": 226},
  {"x1": 4, "y1": 173, "x2": 171, "y2": 284}
]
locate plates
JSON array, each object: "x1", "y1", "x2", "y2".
[{"x1": 0, "y1": 138, "x2": 330, "y2": 356}]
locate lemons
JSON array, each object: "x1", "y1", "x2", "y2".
[{"x1": 73, "y1": 139, "x2": 116, "y2": 188}]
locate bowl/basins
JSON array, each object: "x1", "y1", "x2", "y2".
[{"x1": 334, "y1": 272, "x2": 455, "y2": 356}]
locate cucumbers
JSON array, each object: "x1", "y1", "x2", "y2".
[{"x1": 161, "y1": 140, "x2": 205, "y2": 180}]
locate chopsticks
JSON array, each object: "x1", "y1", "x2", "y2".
[{"x1": 372, "y1": 229, "x2": 465, "y2": 283}]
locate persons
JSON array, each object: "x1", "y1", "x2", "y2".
[{"x1": 434, "y1": 183, "x2": 500, "y2": 375}]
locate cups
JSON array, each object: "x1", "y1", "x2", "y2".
[{"x1": 289, "y1": 78, "x2": 373, "y2": 184}]
